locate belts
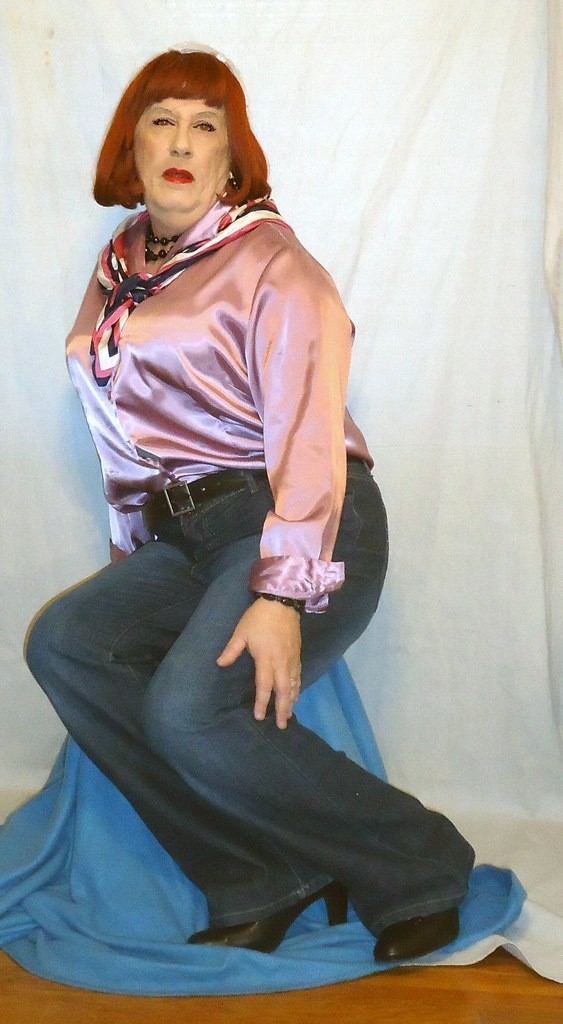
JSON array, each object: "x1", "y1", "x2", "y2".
[{"x1": 149, "y1": 465, "x2": 251, "y2": 526}]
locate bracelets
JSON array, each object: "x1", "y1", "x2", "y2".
[
  {"x1": 255, "y1": 592, "x2": 306, "y2": 615},
  {"x1": 146, "y1": 228, "x2": 181, "y2": 245},
  {"x1": 144, "y1": 246, "x2": 173, "y2": 261}
]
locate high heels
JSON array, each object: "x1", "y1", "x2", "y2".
[
  {"x1": 188, "y1": 880, "x2": 357, "y2": 954},
  {"x1": 376, "y1": 909, "x2": 460, "y2": 963}
]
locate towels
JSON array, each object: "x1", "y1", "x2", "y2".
[
  {"x1": 400, "y1": 900, "x2": 563, "y2": 984},
  {"x1": 0, "y1": 650, "x2": 529, "y2": 1000}
]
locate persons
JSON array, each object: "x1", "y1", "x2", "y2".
[{"x1": 25, "y1": 50, "x2": 477, "y2": 961}]
